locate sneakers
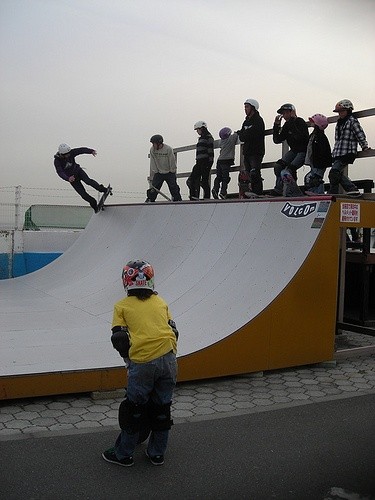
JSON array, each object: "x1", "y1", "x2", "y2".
[
  {"x1": 213, "y1": 193, "x2": 219, "y2": 199},
  {"x1": 219, "y1": 193, "x2": 225, "y2": 200},
  {"x1": 146, "y1": 447, "x2": 165, "y2": 466},
  {"x1": 104, "y1": 188, "x2": 110, "y2": 195},
  {"x1": 102, "y1": 449, "x2": 135, "y2": 467},
  {"x1": 95, "y1": 207, "x2": 99, "y2": 213}
]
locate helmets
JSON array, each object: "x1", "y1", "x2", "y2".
[
  {"x1": 58, "y1": 143, "x2": 72, "y2": 154},
  {"x1": 244, "y1": 98, "x2": 259, "y2": 110},
  {"x1": 332, "y1": 99, "x2": 354, "y2": 112},
  {"x1": 277, "y1": 104, "x2": 296, "y2": 114},
  {"x1": 219, "y1": 127, "x2": 232, "y2": 140},
  {"x1": 150, "y1": 135, "x2": 163, "y2": 145},
  {"x1": 308, "y1": 114, "x2": 328, "y2": 130},
  {"x1": 121, "y1": 260, "x2": 154, "y2": 292},
  {"x1": 194, "y1": 121, "x2": 207, "y2": 130}
]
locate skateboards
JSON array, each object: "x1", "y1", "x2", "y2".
[
  {"x1": 245, "y1": 192, "x2": 274, "y2": 198},
  {"x1": 94, "y1": 184, "x2": 113, "y2": 214},
  {"x1": 149, "y1": 184, "x2": 171, "y2": 202},
  {"x1": 305, "y1": 191, "x2": 362, "y2": 202}
]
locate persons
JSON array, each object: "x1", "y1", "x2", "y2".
[
  {"x1": 186, "y1": 121, "x2": 214, "y2": 200},
  {"x1": 212, "y1": 127, "x2": 238, "y2": 199},
  {"x1": 145, "y1": 135, "x2": 182, "y2": 202},
  {"x1": 265, "y1": 104, "x2": 309, "y2": 198},
  {"x1": 53, "y1": 144, "x2": 108, "y2": 214},
  {"x1": 237, "y1": 99, "x2": 265, "y2": 199},
  {"x1": 102, "y1": 259, "x2": 179, "y2": 468},
  {"x1": 328, "y1": 99, "x2": 368, "y2": 195},
  {"x1": 304, "y1": 114, "x2": 332, "y2": 194}
]
